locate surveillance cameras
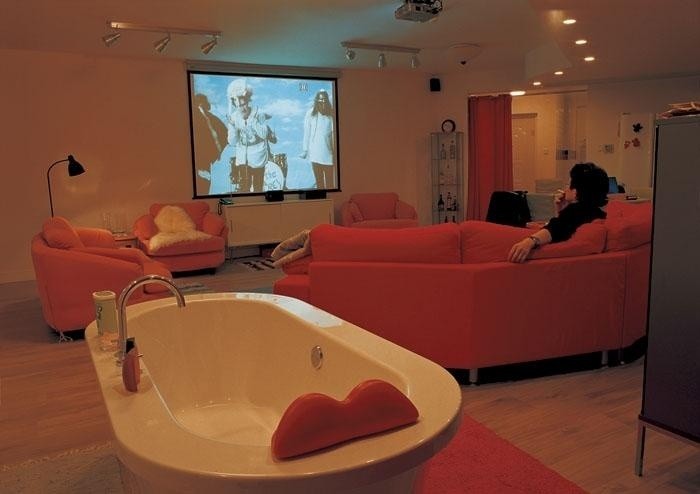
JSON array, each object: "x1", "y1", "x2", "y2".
[{"x1": 460, "y1": 60, "x2": 466, "y2": 64}]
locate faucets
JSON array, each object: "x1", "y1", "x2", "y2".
[{"x1": 116, "y1": 273, "x2": 187, "y2": 361}]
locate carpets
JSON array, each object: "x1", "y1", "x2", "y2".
[
  {"x1": 412, "y1": 408, "x2": 592, "y2": 494},
  {"x1": 1, "y1": 439, "x2": 122, "y2": 494}
]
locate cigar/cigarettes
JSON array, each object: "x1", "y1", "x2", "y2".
[{"x1": 557, "y1": 188, "x2": 566, "y2": 193}]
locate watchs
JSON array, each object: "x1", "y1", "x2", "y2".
[{"x1": 528, "y1": 235, "x2": 540, "y2": 247}]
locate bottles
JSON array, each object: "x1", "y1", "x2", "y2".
[
  {"x1": 444, "y1": 215, "x2": 456, "y2": 223},
  {"x1": 438, "y1": 191, "x2": 457, "y2": 210},
  {"x1": 94, "y1": 290, "x2": 117, "y2": 336},
  {"x1": 441, "y1": 139, "x2": 456, "y2": 158}
]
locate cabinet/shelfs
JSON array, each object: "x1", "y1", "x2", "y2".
[
  {"x1": 219, "y1": 197, "x2": 337, "y2": 264},
  {"x1": 428, "y1": 130, "x2": 467, "y2": 226},
  {"x1": 630, "y1": 107, "x2": 700, "y2": 488}
]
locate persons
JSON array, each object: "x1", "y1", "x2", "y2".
[
  {"x1": 196, "y1": 92, "x2": 228, "y2": 191},
  {"x1": 226, "y1": 77, "x2": 269, "y2": 192},
  {"x1": 300, "y1": 90, "x2": 336, "y2": 188},
  {"x1": 264, "y1": 113, "x2": 276, "y2": 152},
  {"x1": 507, "y1": 161, "x2": 609, "y2": 264}
]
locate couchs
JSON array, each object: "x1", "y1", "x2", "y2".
[
  {"x1": 336, "y1": 191, "x2": 421, "y2": 232},
  {"x1": 30, "y1": 216, "x2": 176, "y2": 343},
  {"x1": 271, "y1": 200, "x2": 653, "y2": 382},
  {"x1": 129, "y1": 198, "x2": 229, "y2": 275}
]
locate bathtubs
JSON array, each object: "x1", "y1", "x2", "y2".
[{"x1": 84, "y1": 292, "x2": 462, "y2": 494}]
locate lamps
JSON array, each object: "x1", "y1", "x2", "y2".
[
  {"x1": 45, "y1": 154, "x2": 87, "y2": 223},
  {"x1": 101, "y1": 18, "x2": 226, "y2": 56},
  {"x1": 338, "y1": 39, "x2": 424, "y2": 72},
  {"x1": 444, "y1": 39, "x2": 482, "y2": 61}
]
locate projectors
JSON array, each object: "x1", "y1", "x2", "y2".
[{"x1": 394, "y1": 2, "x2": 441, "y2": 23}]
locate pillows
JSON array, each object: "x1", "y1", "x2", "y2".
[
  {"x1": 41, "y1": 212, "x2": 86, "y2": 251},
  {"x1": 153, "y1": 204, "x2": 197, "y2": 234}
]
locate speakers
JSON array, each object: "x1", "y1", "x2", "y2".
[{"x1": 430, "y1": 78, "x2": 441, "y2": 91}]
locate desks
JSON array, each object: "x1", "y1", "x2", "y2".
[{"x1": 110, "y1": 229, "x2": 137, "y2": 248}]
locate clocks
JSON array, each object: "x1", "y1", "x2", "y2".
[{"x1": 441, "y1": 117, "x2": 456, "y2": 133}]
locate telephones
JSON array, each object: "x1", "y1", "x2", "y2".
[{"x1": 219, "y1": 198, "x2": 234, "y2": 205}]
